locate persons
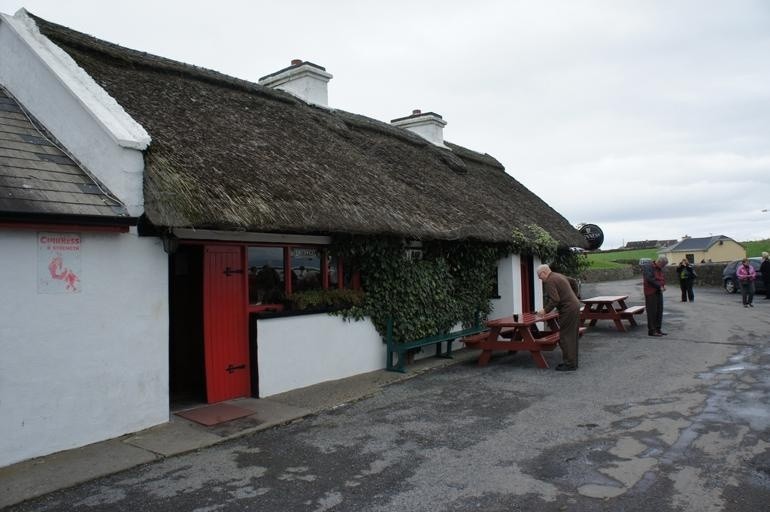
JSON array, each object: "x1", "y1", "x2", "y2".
[
  {"x1": 537, "y1": 264, "x2": 581, "y2": 371},
  {"x1": 759, "y1": 251, "x2": 770, "y2": 300},
  {"x1": 736, "y1": 258, "x2": 756, "y2": 307},
  {"x1": 644, "y1": 255, "x2": 669, "y2": 337},
  {"x1": 675, "y1": 259, "x2": 695, "y2": 302}
]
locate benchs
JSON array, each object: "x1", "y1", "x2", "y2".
[
  {"x1": 459, "y1": 296, "x2": 646, "y2": 370},
  {"x1": 384, "y1": 303, "x2": 493, "y2": 373}
]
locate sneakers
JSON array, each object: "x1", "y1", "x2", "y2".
[
  {"x1": 649, "y1": 331, "x2": 666, "y2": 336},
  {"x1": 556, "y1": 364, "x2": 576, "y2": 371}
]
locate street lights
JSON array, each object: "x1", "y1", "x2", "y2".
[{"x1": 761, "y1": 209, "x2": 770, "y2": 212}]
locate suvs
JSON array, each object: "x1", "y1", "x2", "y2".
[{"x1": 721, "y1": 257, "x2": 765, "y2": 295}]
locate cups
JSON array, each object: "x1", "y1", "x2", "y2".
[{"x1": 513, "y1": 313, "x2": 519, "y2": 322}]
